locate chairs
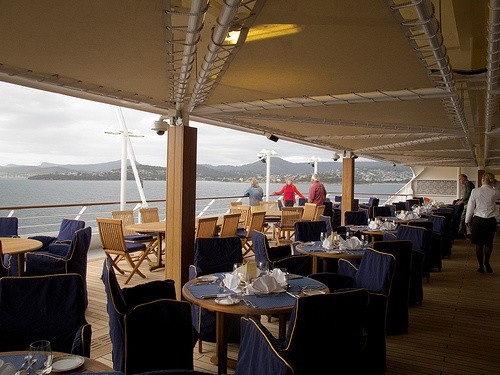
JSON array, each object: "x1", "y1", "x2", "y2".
[{"x1": 0, "y1": 196, "x2": 465, "y2": 375}]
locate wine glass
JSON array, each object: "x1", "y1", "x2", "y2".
[{"x1": 320, "y1": 232, "x2": 327, "y2": 246}]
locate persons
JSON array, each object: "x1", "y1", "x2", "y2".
[
  {"x1": 455, "y1": 174, "x2": 474, "y2": 238},
  {"x1": 308, "y1": 174, "x2": 327, "y2": 207},
  {"x1": 244, "y1": 179, "x2": 264, "y2": 206},
  {"x1": 272, "y1": 178, "x2": 306, "y2": 207},
  {"x1": 466, "y1": 172, "x2": 500, "y2": 274}
]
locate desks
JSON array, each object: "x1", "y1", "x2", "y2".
[
  {"x1": 125, "y1": 217, "x2": 245, "y2": 272},
  {"x1": 375, "y1": 216, "x2": 428, "y2": 223},
  {"x1": 182, "y1": 271, "x2": 329, "y2": 370},
  {"x1": 350, "y1": 226, "x2": 397, "y2": 243},
  {"x1": 296, "y1": 240, "x2": 373, "y2": 274},
  {"x1": 265, "y1": 210, "x2": 300, "y2": 240},
  {"x1": 0, "y1": 351, "x2": 114, "y2": 375},
  {"x1": 0, "y1": 238, "x2": 43, "y2": 276}
]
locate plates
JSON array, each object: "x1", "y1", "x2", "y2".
[
  {"x1": 303, "y1": 289, "x2": 325, "y2": 296},
  {"x1": 198, "y1": 276, "x2": 219, "y2": 281},
  {"x1": 214, "y1": 297, "x2": 240, "y2": 305},
  {"x1": 50, "y1": 354, "x2": 86, "y2": 372}
]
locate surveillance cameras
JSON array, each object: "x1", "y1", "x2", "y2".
[
  {"x1": 331, "y1": 154, "x2": 340, "y2": 161},
  {"x1": 150, "y1": 121, "x2": 169, "y2": 135}
]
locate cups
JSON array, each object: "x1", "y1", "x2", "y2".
[
  {"x1": 27, "y1": 340, "x2": 52, "y2": 375},
  {"x1": 256, "y1": 261, "x2": 270, "y2": 278},
  {"x1": 232, "y1": 263, "x2": 244, "y2": 272}
]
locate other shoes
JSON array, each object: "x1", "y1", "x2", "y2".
[
  {"x1": 477, "y1": 265, "x2": 484, "y2": 273},
  {"x1": 484, "y1": 261, "x2": 493, "y2": 273}
]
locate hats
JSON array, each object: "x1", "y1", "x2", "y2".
[{"x1": 312, "y1": 174, "x2": 320, "y2": 182}]
